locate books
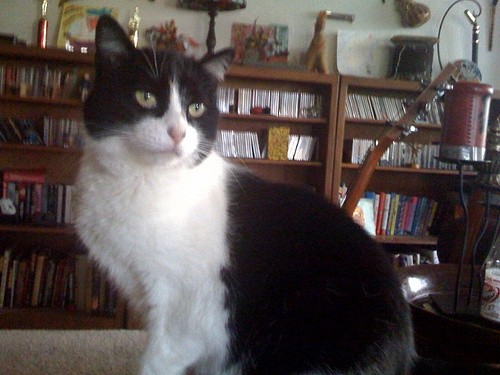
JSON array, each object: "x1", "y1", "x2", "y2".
[
  {"x1": 1, "y1": 114, "x2": 88, "y2": 148},
  {"x1": 1, "y1": 61, "x2": 95, "y2": 101},
  {"x1": 345, "y1": 136, "x2": 480, "y2": 174},
  {"x1": 215, "y1": 86, "x2": 329, "y2": 120},
  {"x1": 0, "y1": 248, "x2": 116, "y2": 315},
  {"x1": 212, "y1": 125, "x2": 323, "y2": 162},
  {"x1": 345, "y1": 92, "x2": 446, "y2": 128},
  {"x1": 4, "y1": 167, "x2": 44, "y2": 182},
  {"x1": 338, "y1": 184, "x2": 439, "y2": 237},
  {"x1": 0, "y1": 181, "x2": 76, "y2": 226},
  {"x1": 388, "y1": 249, "x2": 439, "y2": 267}
]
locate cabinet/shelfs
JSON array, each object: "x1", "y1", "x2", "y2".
[
  {"x1": 332, "y1": 73, "x2": 499, "y2": 264},
  {"x1": 0, "y1": 43, "x2": 126, "y2": 332},
  {"x1": 126, "y1": 63, "x2": 341, "y2": 330}
]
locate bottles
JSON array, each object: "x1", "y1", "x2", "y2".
[{"x1": 479, "y1": 235, "x2": 500, "y2": 325}]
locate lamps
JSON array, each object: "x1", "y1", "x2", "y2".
[{"x1": 175, "y1": 0, "x2": 247, "y2": 66}]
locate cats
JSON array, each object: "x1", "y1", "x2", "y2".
[{"x1": 69, "y1": 12, "x2": 419, "y2": 375}]
